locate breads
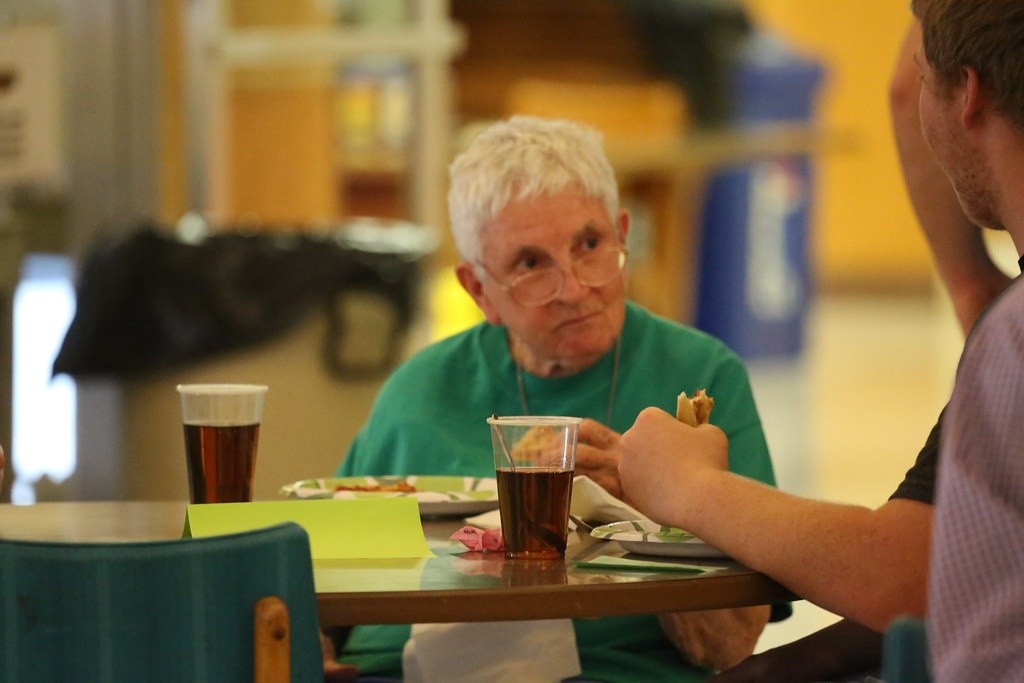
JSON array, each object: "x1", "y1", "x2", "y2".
[{"x1": 677, "y1": 389, "x2": 714, "y2": 427}]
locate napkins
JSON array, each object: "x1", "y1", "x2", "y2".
[{"x1": 464, "y1": 475, "x2": 662, "y2": 537}]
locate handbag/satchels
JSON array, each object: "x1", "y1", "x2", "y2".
[{"x1": 50, "y1": 225, "x2": 414, "y2": 382}]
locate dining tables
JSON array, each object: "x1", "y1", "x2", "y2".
[{"x1": 316, "y1": 512, "x2": 796, "y2": 627}]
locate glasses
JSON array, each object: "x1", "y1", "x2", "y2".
[{"x1": 479, "y1": 223, "x2": 629, "y2": 306}]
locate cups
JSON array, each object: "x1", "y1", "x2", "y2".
[
  {"x1": 175, "y1": 384, "x2": 270, "y2": 504},
  {"x1": 487, "y1": 416, "x2": 582, "y2": 562}
]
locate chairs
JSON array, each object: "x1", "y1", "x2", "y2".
[{"x1": 0, "y1": 504, "x2": 327, "y2": 683}]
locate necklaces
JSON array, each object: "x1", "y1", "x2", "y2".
[{"x1": 517, "y1": 337, "x2": 620, "y2": 429}]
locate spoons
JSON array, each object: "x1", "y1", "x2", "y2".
[
  {"x1": 570, "y1": 515, "x2": 593, "y2": 533},
  {"x1": 492, "y1": 414, "x2": 564, "y2": 552}
]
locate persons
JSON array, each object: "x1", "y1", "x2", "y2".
[
  {"x1": 320, "y1": 117, "x2": 792, "y2": 683},
  {"x1": 618, "y1": 0, "x2": 1024, "y2": 682}
]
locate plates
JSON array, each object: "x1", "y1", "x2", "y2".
[
  {"x1": 278, "y1": 475, "x2": 500, "y2": 516},
  {"x1": 589, "y1": 520, "x2": 729, "y2": 559}
]
